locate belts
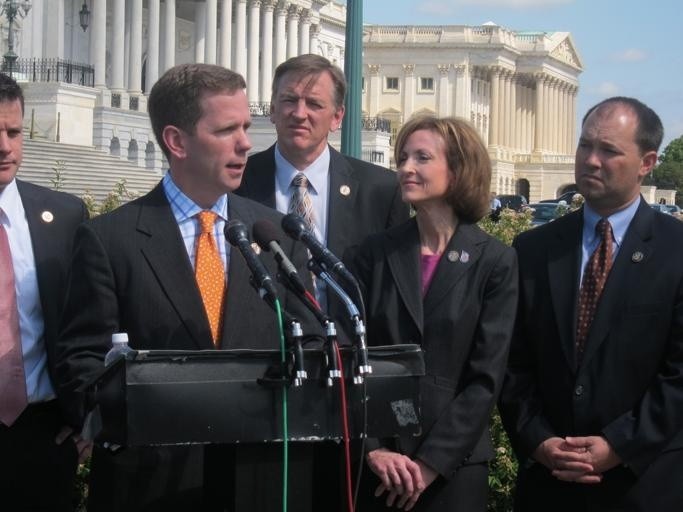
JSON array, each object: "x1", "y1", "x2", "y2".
[{"x1": 404, "y1": 423, "x2": 422, "y2": 438}]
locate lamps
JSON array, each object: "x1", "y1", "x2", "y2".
[{"x1": 79, "y1": 0, "x2": 90, "y2": 32}]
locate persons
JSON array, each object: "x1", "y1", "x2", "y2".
[
  {"x1": 330, "y1": 113, "x2": 519, "y2": 510},
  {"x1": 0, "y1": 71, "x2": 102, "y2": 512},
  {"x1": 227, "y1": 53, "x2": 410, "y2": 510},
  {"x1": 51, "y1": 61, "x2": 359, "y2": 509},
  {"x1": 494, "y1": 96, "x2": 682, "y2": 510},
  {"x1": 489, "y1": 190, "x2": 682, "y2": 225}
]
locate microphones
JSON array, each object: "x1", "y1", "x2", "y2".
[
  {"x1": 248, "y1": 274, "x2": 299, "y2": 329},
  {"x1": 251, "y1": 218, "x2": 307, "y2": 294},
  {"x1": 280, "y1": 213, "x2": 358, "y2": 287},
  {"x1": 305, "y1": 257, "x2": 362, "y2": 321},
  {"x1": 223, "y1": 219, "x2": 277, "y2": 296},
  {"x1": 276, "y1": 272, "x2": 332, "y2": 326}
]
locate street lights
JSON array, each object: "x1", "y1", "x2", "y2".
[{"x1": 0, "y1": 0, "x2": 32, "y2": 78}]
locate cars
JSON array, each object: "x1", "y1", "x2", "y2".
[
  {"x1": 649, "y1": 204, "x2": 683, "y2": 215},
  {"x1": 491, "y1": 191, "x2": 576, "y2": 226}
]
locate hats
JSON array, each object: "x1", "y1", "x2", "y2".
[{"x1": 191, "y1": 209, "x2": 225, "y2": 346}]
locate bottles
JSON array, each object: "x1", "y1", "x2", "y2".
[{"x1": 103, "y1": 332, "x2": 136, "y2": 367}]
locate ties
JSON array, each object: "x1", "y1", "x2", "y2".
[
  {"x1": 570, "y1": 219, "x2": 615, "y2": 358},
  {"x1": 286, "y1": 173, "x2": 322, "y2": 295},
  {"x1": 0, "y1": 211, "x2": 28, "y2": 429}
]
